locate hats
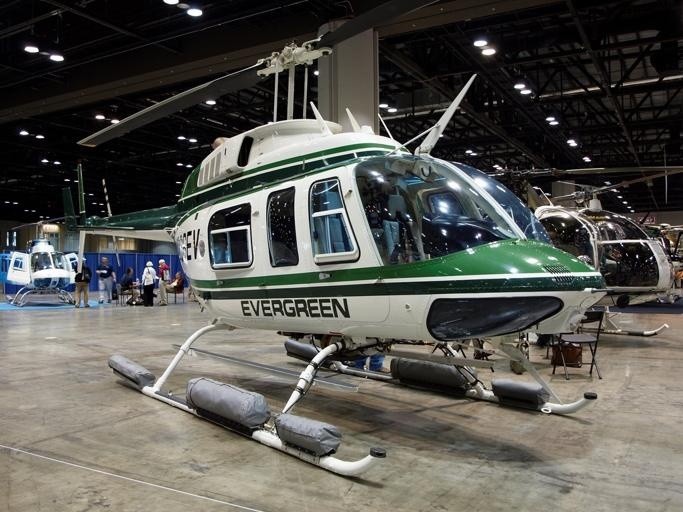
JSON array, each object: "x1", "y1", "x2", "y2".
[
  {"x1": 82, "y1": 257, "x2": 87, "y2": 261},
  {"x1": 146, "y1": 261, "x2": 154, "y2": 266}
]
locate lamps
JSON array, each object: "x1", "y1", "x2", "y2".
[
  {"x1": 175, "y1": 153, "x2": 195, "y2": 170},
  {"x1": 471, "y1": 31, "x2": 498, "y2": 58},
  {"x1": 176, "y1": 127, "x2": 199, "y2": 143},
  {"x1": 39, "y1": 151, "x2": 63, "y2": 166},
  {"x1": 91, "y1": 108, "x2": 121, "y2": 125},
  {"x1": 163, "y1": 0, "x2": 204, "y2": 18},
  {"x1": 16, "y1": 124, "x2": 47, "y2": 140},
  {"x1": 22, "y1": 33, "x2": 65, "y2": 63}
]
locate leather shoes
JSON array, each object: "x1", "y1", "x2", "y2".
[
  {"x1": 75, "y1": 305, "x2": 80, "y2": 308},
  {"x1": 135, "y1": 301, "x2": 143, "y2": 306},
  {"x1": 98, "y1": 300, "x2": 104, "y2": 303},
  {"x1": 107, "y1": 299, "x2": 112, "y2": 303},
  {"x1": 126, "y1": 301, "x2": 135, "y2": 306},
  {"x1": 84, "y1": 305, "x2": 90, "y2": 307}
]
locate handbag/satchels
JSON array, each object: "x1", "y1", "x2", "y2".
[
  {"x1": 161, "y1": 269, "x2": 170, "y2": 281},
  {"x1": 143, "y1": 268, "x2": 154, "y2": 286},
  {"x1": 112, "y1": 280, "x2": 118, "y2": 300}
]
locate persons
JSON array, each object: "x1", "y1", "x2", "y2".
[
  {"x1": 72, "y1": 255, "x2": 198, "y2": 309},
  {"x1": 347, "y1": 355, "x2": 392, "y2": 374}
]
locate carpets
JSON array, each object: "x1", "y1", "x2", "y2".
[{"x1": 0, "y1": 298, "x2": 110, "y2": 311}]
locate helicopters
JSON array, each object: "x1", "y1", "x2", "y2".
[{"x1": 4, "y1": 215, "x2": 80, "y2": 308}]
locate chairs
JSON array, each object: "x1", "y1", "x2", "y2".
[
  {"x1": 551, "y1": 311, "x2": 608, "y2": 381},
  {"x1": 114, "y1": 279, "x2": 186, "y2": 307},
  {"x1": 316, "y1": 190, "x2": 416, "y2": 267}
]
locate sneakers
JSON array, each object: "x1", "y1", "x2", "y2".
[{"x1": 158, "y1": 300, "x2": 167, "y2": 306}]
